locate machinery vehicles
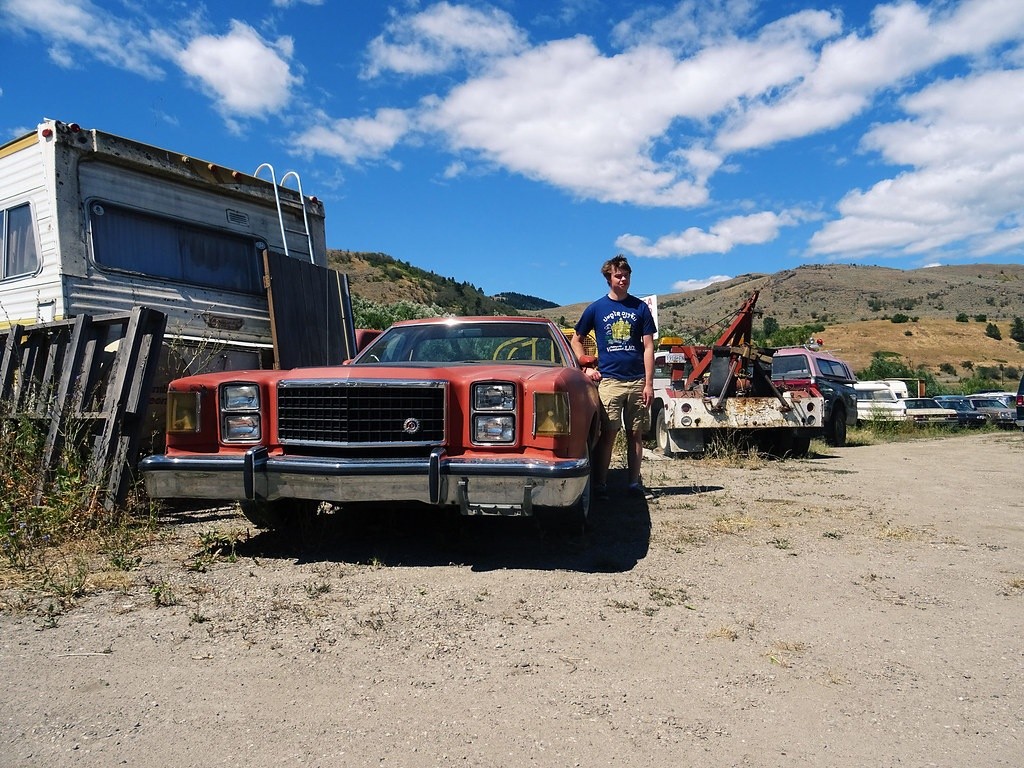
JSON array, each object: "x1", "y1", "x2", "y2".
[{"x1": 617, "y1": 289, "x2": 830, "y2": 459}]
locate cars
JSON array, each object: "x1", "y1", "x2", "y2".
[
  {"x1": 136, "y1": 314, "x2": 604, "y2": 541},
  {"x1": 844, "y1": 372, "x2": 1024, "y2": 433}
]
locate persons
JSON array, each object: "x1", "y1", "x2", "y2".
[{"x1": 571, "y1": 256, "x2": 657, "y2": 500}]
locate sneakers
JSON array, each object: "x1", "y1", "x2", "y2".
[
  {"x1": 629, "y1": 484, "x2": 654, "y2": 500},
  {"x1": 595, "y1": 484, "x2": 609, "y2": 500}
]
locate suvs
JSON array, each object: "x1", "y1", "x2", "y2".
[{"x1": 745, "y1": 343, "x2": 857, "y2": 448}]
